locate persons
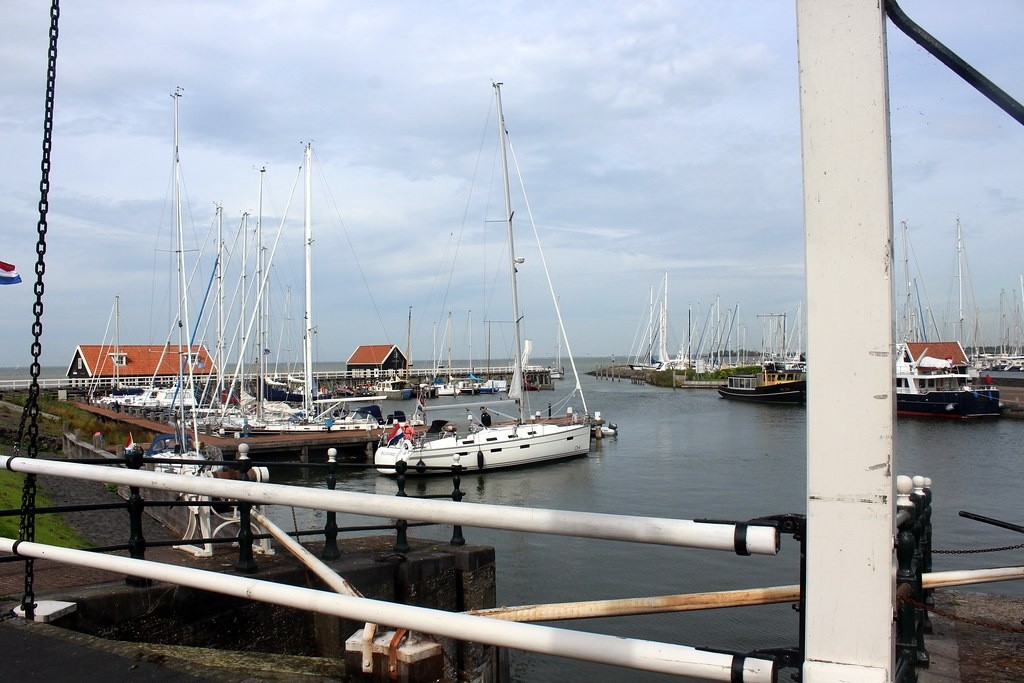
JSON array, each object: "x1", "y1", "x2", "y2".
[
  {"x1": 404, "y1": 422, "x2": 418, "y2": 440},
  {"x1": 481, "y1": 410, "x2": 491, "y2": 427}
]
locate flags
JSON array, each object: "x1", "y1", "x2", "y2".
[
  {"x1": 125, "y1": 433, "x2": 133, "y2": 450},
  {"x1": 0, "y1": 260, "x2": 23, "y2": 285},
  {"x1": 388, "y1": 423, "x2": 403, "y2": 449}
]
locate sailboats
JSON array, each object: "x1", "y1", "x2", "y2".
[
  {"x1": 89, "y1": 78, "x2": 620, "y2": 479},
  {"x1": 631, "y1": 238, "x2": 1023, "y2": 421}
]
